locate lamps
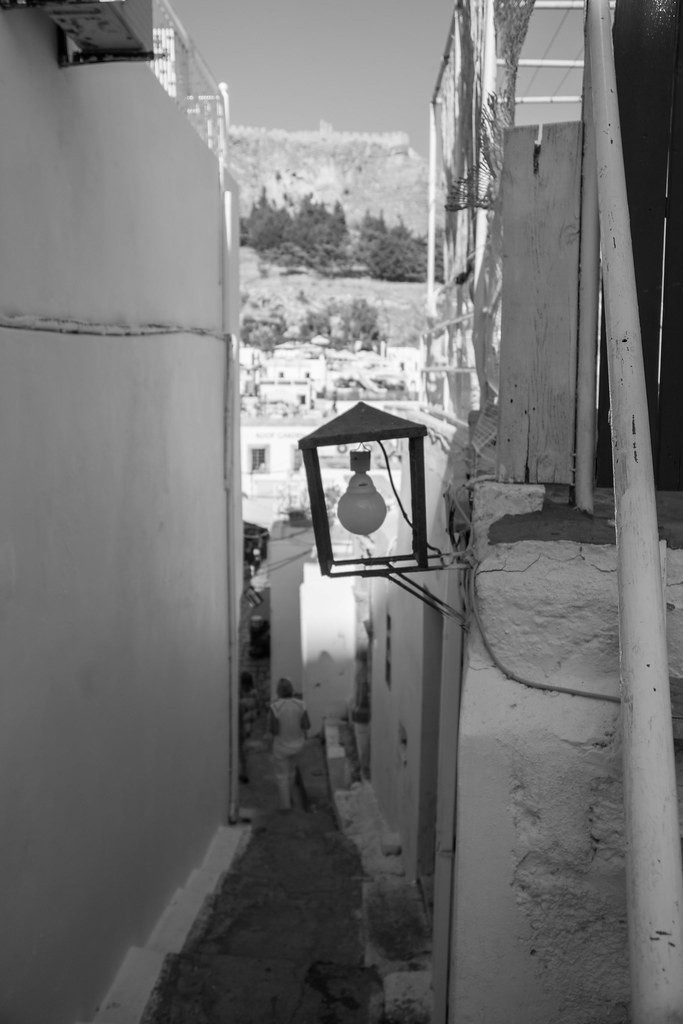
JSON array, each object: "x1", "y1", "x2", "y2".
[{"x1": 299, "y1": 404, "x2": 432, "y2": 573}]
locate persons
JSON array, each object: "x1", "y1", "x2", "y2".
[
  {"x1": 337, "y1": 650, "x2": 372, "y2": 791},
  {"x1": 267, "y1": 677, "x2": 323, "y2": 812},
  {"x1": 235, "y1": 669, "x2": 256, "y2": 784}
]
